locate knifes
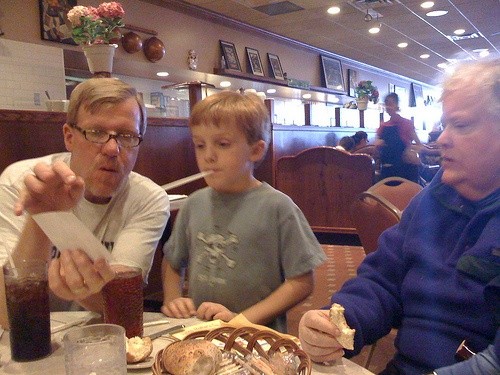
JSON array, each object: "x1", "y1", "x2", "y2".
[{"x1": 143, "y1": 324, "x2": 185, "y2": 340}]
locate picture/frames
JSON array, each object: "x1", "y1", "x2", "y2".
[
  {"x1": 412, "y1": 82, "x2": 424, "y2": 107},
  {"x1": 267, "y1": 53, "x2": 284, "y2": 80},
  {"x1": 320, "y1": 54, "x2": 345, "y2": 91},
  {"x1": 348, "y1": 69, "x2": 357, "y2": 97},
  {"x1": 220, "y1": 40, "x2": 242, "y2": 73},
  {"x1": 38, "y1": 0, "x2": 77, "y2": 44},
  {"x1": 245, "y1": 47, "x2": 265, "y2": 77}
]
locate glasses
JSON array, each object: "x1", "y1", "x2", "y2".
[{"x1": 68, "y1": 122, "x2": 144, "y2": 148}]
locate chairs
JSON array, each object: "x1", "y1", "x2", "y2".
[{"x1": 352, "y1": 176, "x2": 424, "y2": 255}]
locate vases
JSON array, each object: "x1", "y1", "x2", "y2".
[
  {"x1": 82, "y1": 44, "x2": 115, "y2": 73},
  {"x1": 356, "y1": 99, "x2": 368, "y2": 110}
]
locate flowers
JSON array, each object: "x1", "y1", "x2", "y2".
[
  {"x1": 354, "y1": 81, "x2": 379, "y2": 104},
  {"x1": 67, "y1": 1, "x2": 125, "y2": 46}
]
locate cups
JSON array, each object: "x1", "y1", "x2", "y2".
[
  {"x1": 45, "y1": 101, "x2": 65, "y2": 113},
  {"x1": 62, "y1": 323, "x2": 127, "y2": 375},
  {"x1": 2, "y1": 259, "x2": 52, "y2": 363},
  {"x1": 100, "y1": 267, "x2": 144, "y2": 339}
]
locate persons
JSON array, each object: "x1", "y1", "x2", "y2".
[
  {"x1": 299, "y1": 56, "x2": 500, "y2": 375},
  {"x1": 334, "y1": 93, "x2": 442, "y2": 186},
  {"x1": 0, "y1": 78, "x2": 170, "y2": 328},
  {"x1": 162, "y1": 91, "x2": 328, "y2": 333}
]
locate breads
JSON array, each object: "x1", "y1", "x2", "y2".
[
  {"x1": 162, "y1": 339, "x2": 223, "y2": 375},
  {"x1": 329, "y1": 303, "x2": 355, "y2": 350},
  {"x1": 123, "y1": 336, "x2": 152, "y2": 364}
]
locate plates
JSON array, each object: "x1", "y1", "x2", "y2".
[{"x1": 126, "y1": 335, "x2": 181, "y2": 369}]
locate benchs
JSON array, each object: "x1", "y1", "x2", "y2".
[{"x1": 276, "y1": 146, "x2": 373, "y2": 235}]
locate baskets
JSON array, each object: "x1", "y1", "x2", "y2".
[{"x1": 152, "y1": 325, "x2": 312, "y2": 375}]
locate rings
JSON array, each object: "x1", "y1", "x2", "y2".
[{"x1": 70, "y1": 286, "x2": 87, "y2": 294}]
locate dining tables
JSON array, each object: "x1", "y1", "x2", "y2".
[{"x1": 0, "y1": 311, "x2": 375, "y2": 375}]
